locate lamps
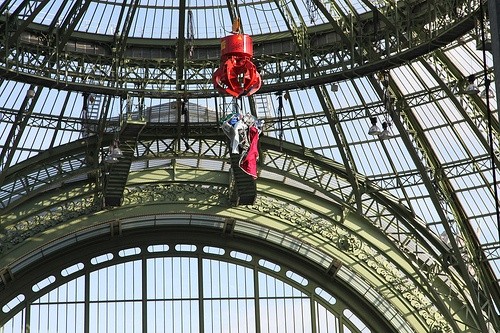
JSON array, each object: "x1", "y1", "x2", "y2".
[
  {"x1": 368, "y1": 116, "x2": 383, "y2": 135},
  {"x1": 103, "y1": 144, "x2": 120, "y2": 162},
  {"x1": 480, "y1": 79, "x2": 495, "y2": 98},
  {"x1": 379, "y1": 121, "x2": 393, "y2": 139},
  {"x1": 110, "y1": 137, "x2": 124, "y2": 158},
  {"x1": 466, "y1": 74, "x2": 481, "y2": 94}
]
